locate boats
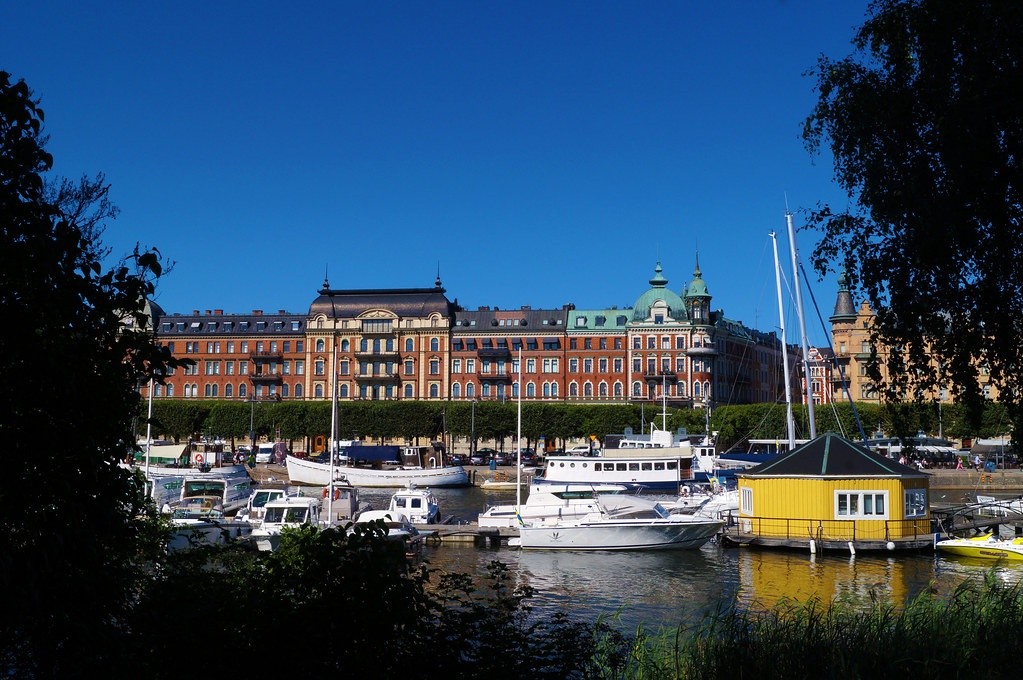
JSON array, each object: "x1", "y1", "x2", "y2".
[
  {"x1": 518, "y1": 481, "x2": 727, "y2": 550},
  {"x1": 136, "y1": 210, "x2": 869, "y2": 552},
  {"x1": 935, "y1": 529, "x2": 1023, "y2": 561},
  {"x1": 959, "y1": 496, "x2": 1023, "y2": 518}
]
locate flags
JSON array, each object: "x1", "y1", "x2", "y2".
[
  {"x1": 516, "y1": 511, "x2": 524, "y2": 525},
  {"x1": 708, "y1": 475, "x2": 726, "y2": 488}
]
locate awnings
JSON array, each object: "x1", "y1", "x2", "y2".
[{"x1": 890, "y1": 445, "x2": 957, "y2": 453}]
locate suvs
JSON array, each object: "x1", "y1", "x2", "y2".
[{"x1": 565, "y1": 445, "x2": 591, "y2": 457}]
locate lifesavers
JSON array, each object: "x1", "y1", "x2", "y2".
[
  {"x1": 195, "y1": 454, "x2": 203, "y2": 462},
  {"x1": 709, "y1": 477, "x2": 719, "y2": 485},
  {"x1": 323, "y1": 486, "x2": 340, "y2": 501},
  {"x1": 681, "y1": 486, "x2": 690, "y2": 493}
]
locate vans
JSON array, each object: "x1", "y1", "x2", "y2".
[{"x1": 256, "y1": 442, "x2": 276, "y2": 464}]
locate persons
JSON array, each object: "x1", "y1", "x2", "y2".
[
  {"x1": 162, "y1": 501, "x2": 171, "y2": 513},
  {"x1": 955, "y1": 457, "x2": 966, "y2": 471},
  {"x1": 974, "y1": 456, "x2": 981, "y2": 472},
  {"x1": 899, "y1": 455, "x2": 929, "y2": 471}
]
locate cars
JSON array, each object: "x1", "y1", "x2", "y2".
[
  {"x1": 511, "y1": 447, "x2": 533, "y2": 462},
  {"x1": 493, "y1": 452, "x2": 513, "y2": 467},
  {"x1": 520, "y1": 451, "x2": 539, "y2": 467},
  {"x1": 542, "y1": 448, "x2": 567, "y2": 456},
  {"x1": 317, "y1": 451, "x2": 331, "y2": 464},
  {"x1": 450, "y1": 453, "x2": 468, "y2": 467},
  {"x1": 472, "y1": 447, "x2": 498, "y2": 457},
  {"x1": 220, "y1": 452, "x2": 234, "y2": 464},
  {"x1": 469, "y1": 453, "x2": 490, "y2": 466}
]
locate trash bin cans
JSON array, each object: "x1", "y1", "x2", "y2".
[
  {"x1": 489, "y1": 460, "x2": 497, "y2": 470},
  {"x1": 984, "y1": 462, "x2": 995, "y2": 472}
]
